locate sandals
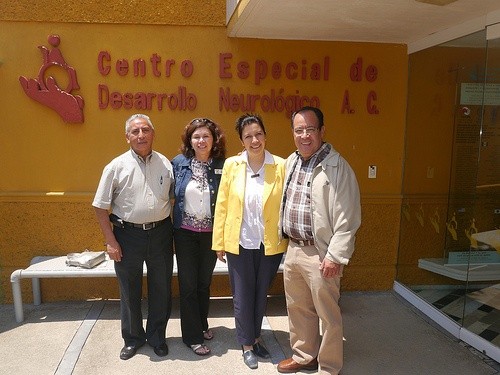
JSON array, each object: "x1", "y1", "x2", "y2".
[
  {"x1": 203, "y1": 328, "x2": 214, "y2": 339},
  {"x1": 188, "y1": 342, "x2": 210, "y2": 355}
]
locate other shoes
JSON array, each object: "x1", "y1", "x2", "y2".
[
  {"x1": 147, "y1": 339, "x2": 169, "y2": 357},
  {"x1": 241, "y1": 349, "x2": 258, "y2": 369},
  {"x1": 120, "y1": 339, "x2": 146, "y2": 360},
  {"x1": 253, "y1": 343, "x2": 271, "y2": 359}
]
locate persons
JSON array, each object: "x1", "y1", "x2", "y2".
[
  {"x1": 92, "y1": 113, "x2": 175, "y2": 360},
  {"x1": 237, "y1": 105, "x2": 361, "y2": 375},
  {"x1": 169, "y1": 118, "x2": 228, "y2": 355},
  {"x1": 212, "y1": 112, "x2": 286, "y2": 369}
]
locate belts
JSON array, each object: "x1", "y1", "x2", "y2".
[
  {"x1": 124, "y1": 217, "x2": 170, "y2": 231},
  {"x1": 290, "y1": 237, "x2": 314, "y2": 247}
]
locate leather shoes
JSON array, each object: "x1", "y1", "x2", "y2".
[{"x1": 277, "y1": 357, "x2": 318, "y2": 373}]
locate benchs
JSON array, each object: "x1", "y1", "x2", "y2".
[{"x1": 11, "y1": 256, "x2": 286, "y2": 321}]
